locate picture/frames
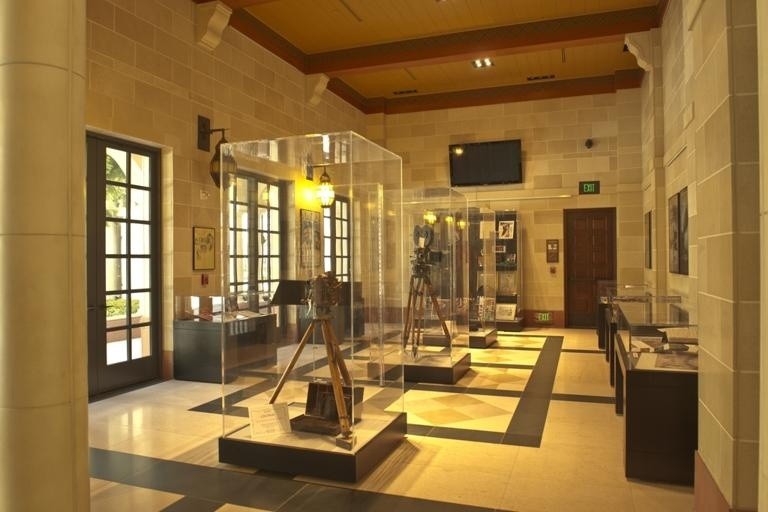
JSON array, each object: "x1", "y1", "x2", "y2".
[
  {"x1": 299, "y1": 208, "x2": 322, "y2": 269},
  {"x1": 192, "y1": 225, "x2": 216, "y2": 272},
  {"x1": 495, "y1": 303, "x2": 517, "y2": 321},
  {"x1": 497, "y1": 219, "x2": 514, "y2": 240},
  {"x1": 667, "y1": 185, "x2": 689, "y2": 275}
]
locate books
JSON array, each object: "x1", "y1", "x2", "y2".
[
  {"x1": 630, "y1": 341, "x2": 689, "y2": 352},
  {"x1": 665, "y1": 328, "x2": 698, "y2": 343}
]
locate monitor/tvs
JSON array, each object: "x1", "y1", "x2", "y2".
[{"x1": 449, "y1": 139, "x2": 522, "y2": 187}]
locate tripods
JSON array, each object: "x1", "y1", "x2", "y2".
[
  {"x1": 403, "y1": 273, "x2": 452, "y2": 359},
  {"x1": 269, "y1": 316, "x2": 353, "y2": 440}
]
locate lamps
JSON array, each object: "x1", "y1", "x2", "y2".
[
  {"x1": 196, "y1": 114, "x2": 237, "y2": 193},
  {"x1": 305, "y1": 151, "x2": 335, "y2": 209}
]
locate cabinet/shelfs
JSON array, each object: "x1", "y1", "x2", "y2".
[{"x1": 469, "y1": 213, "x2": 525, "y2": 333}]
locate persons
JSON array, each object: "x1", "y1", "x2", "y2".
[{"x1": 501, "y1": 223, "x2": 510, "y2": 237}]
